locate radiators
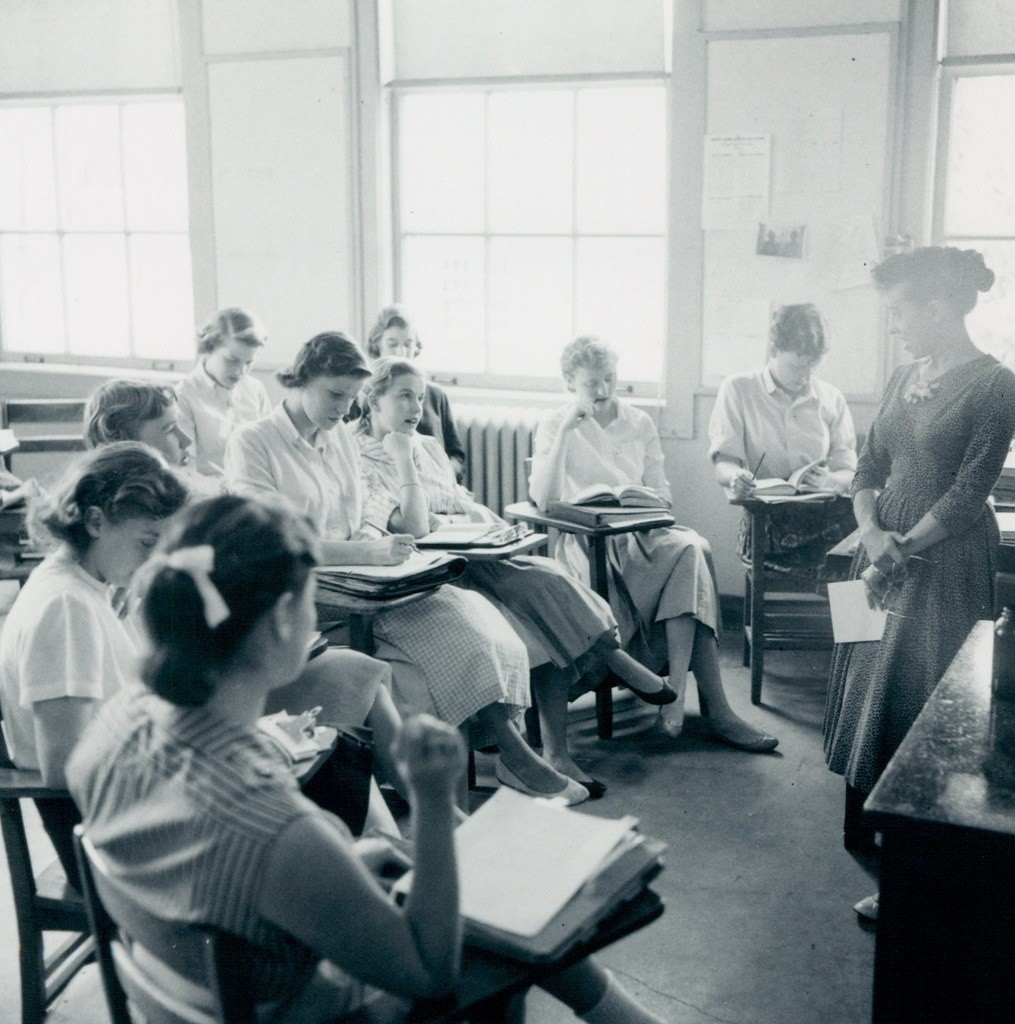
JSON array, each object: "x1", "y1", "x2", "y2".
[{"x1": 442, "y1": 404, "x2": 550, "y2": 530}]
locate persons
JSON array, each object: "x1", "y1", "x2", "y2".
[
  {"x1": 225, "y1": 330, "x2": 591, "y2": 807},
  {"x1": 0, "y1": 453, "x2": 30, "y2": 510},
  {"x1": 820, "y1": 247, "x2": 1015, "y2": 920},
  {"x1": 80, "y1": 378, "x2": 469, "y2": 840},
  {"x1": 68, "y1": 493, "x2": 665, "y2": 1023},
  {"x1": 529, "y1": 334, "x2": 780, "y2": 752},
  {"x1": 1, "y1": 441, "x2": 191, "y2": 789},
  {"x1": 709, "y1": 303, "x2": 858, "y2": 576},
  {"x1": 345, "y1": 304, "x2": 466, "y2": 483},
  {"x1": 176, "y1": 309, "x2": 271, "y2": 479},
  {"x1": 350, "y1": 356, "x2": 677, "y2": 798}
]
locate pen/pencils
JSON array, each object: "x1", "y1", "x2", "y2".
[
  {"x1": 751, "y1": 449, "x2": 768, "y2": 481},
  {"x1": 364, "y1": 520, "x2": 424, "y2": 556},
  {"x1": 580, "y1": 399, "x2": 598, "y2": 418}
]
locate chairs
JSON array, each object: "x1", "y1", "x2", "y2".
[{"x1": 1, "y1": 396, "x2": 254, "y2": 1024}]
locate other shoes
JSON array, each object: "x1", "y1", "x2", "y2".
[
  {"x1": 854, "y1": 891, "x2": 876, "y2": 918},
  {"x1": 498, "y1": 756, "x2": 588, "y2": 807}
]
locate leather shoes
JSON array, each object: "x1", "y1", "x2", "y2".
[
  {"x1": 710, "y1": 720, "x2": 779, "y2": 751},
  {"x1": 606, "y1": 660, "x2": 677, "y2": 704},
  {"x1": 583, "y1": 778, "x2": 605, "y2": 794}
]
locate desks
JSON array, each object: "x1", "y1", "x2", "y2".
[{"x1": 311, "y1": 488, "x2": 1015, "y2": 1024}]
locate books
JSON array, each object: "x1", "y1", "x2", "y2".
[
  {"x1": 414, "y1": 522, "x2": 534, "y2": 546},
  {"x1": 570, "y1": 479, "x2": 669, "y2": 508},
  {"x1": 548, "y1": 500, "x2": 671, "y2": 528},
  {"x1": 315, "y1": 550, "x2": 470, "y2": 598},
  {"x1": 393, "y1": 786, "x2": 670, "y2": 961},
  {"x1": 750, "y1": 458, "x2": 823, "y2": 496}
]
